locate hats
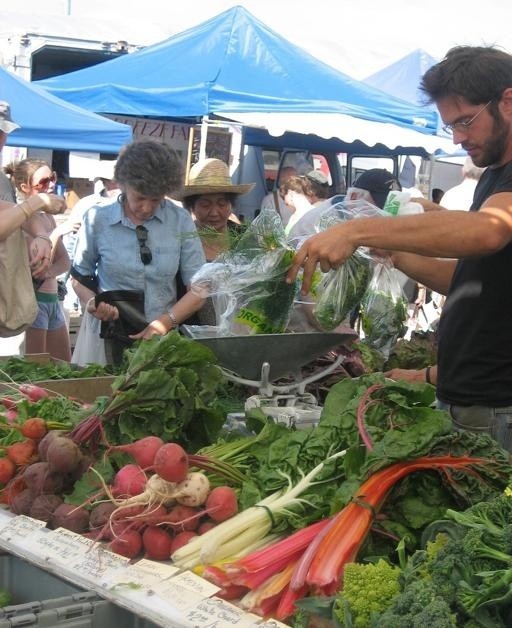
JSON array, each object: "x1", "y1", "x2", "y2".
[
  {"x1": 305, "y1": 168, "x2": 328, "y2": 185},
  {"x1": 167, "y1": 157, "x2": 256, "y2": 202},
  {"x1": 353, "y1": 169, "x2": 402, "y2": 210},
  {"x1": 0, "y1": 101, "x2": 21, "y2": 134}
]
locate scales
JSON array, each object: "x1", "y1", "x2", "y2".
[{"x1": 193, "y1": 331, "x2": 359, "y2": 436}]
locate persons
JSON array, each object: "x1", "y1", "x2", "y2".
[
  {"x1": 264, "y1": 158, "x2": 486, "y2": 335},
  {"x1": 0, "y1": 101, "x2": 251, "y2": 372},
  {"x1": 282, "y1": 42, "x2": 512, "y2": 457}
]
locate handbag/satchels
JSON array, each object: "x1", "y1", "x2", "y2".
[
  {"x1": 95, "y1": 289, "x2": 149, "y2": 368},
  {"x1": 404, "y1": 303, "x2": 431, "y2": 341}
]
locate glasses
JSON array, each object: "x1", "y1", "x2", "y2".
[
  {"x1": 31, "y1": 171, "x2": 58, "y2": 192},
  {"x1": 442, "y1": 100, "x2": 490, "y2": 135},
  {"x1": 136, "y1": 225, "x2": 152, "y2": 264}
]
